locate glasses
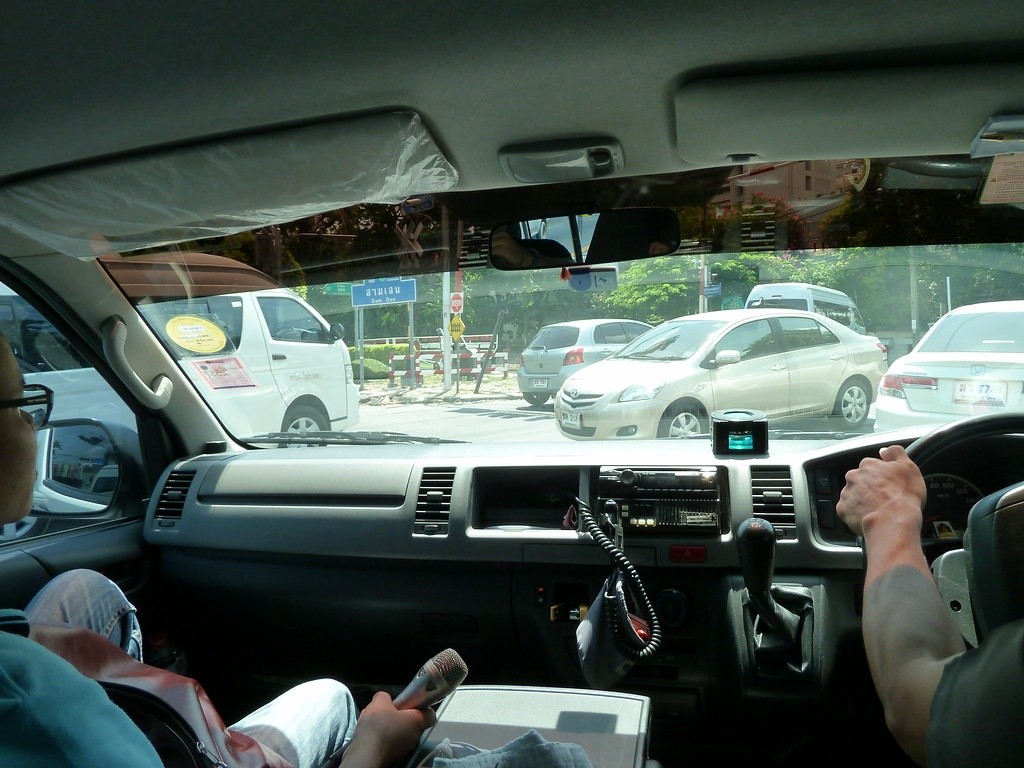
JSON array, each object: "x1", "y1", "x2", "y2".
[{"x1": 0, "y1": 383, "x2": 55, "y2": 430}]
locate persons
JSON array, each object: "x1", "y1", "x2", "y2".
[
  {"x1": 492, "y1": 222, "x2": 576, "y2": 267},
  {"x1": 0, "y1": 333, "x2": 437, "y2": 768},
  {"x1": 836, "y1": 444, "x2": 1024, "y2": 768}
]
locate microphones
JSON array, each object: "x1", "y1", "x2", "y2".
[{"x1": 331, "y1": 648, "x2": 469, "y2": 764}]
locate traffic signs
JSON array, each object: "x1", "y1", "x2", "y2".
[{"x1": 323, "y1": 284, "x2": 356, "y2": 297}]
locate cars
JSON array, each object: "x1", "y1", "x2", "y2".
[
  {"x1": 875, "y1": 299, "x2": 1024, "y2": 446},
  {"x1": 553, "y1": 306, "x2": 890, "y2": 442},
  {"x1": 518, "y1": 315, "x2": 655, "y2": 404}
]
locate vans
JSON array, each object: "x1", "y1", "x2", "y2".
[
  {"x1": 744, "y1": 280, "x2": 867, "y2": 336},
  {"x1": 0, "y1": 278, "x2": 358, "y2": 493}
]
